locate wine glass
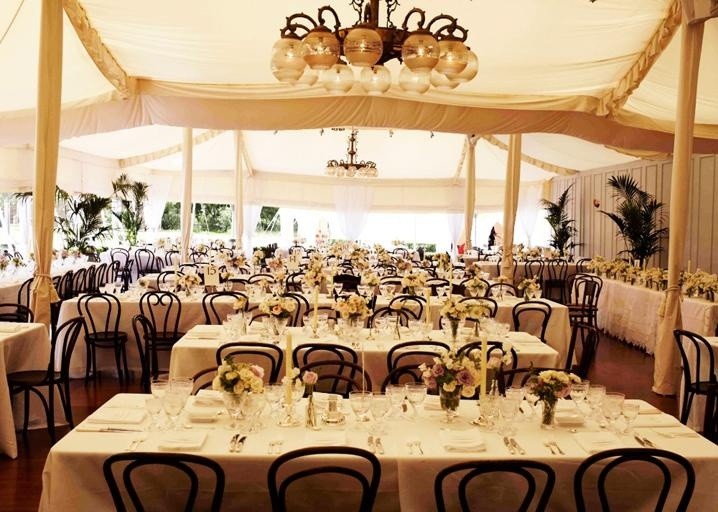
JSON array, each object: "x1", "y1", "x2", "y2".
[
  {"x1": 97, "y1": 243, "x2": 584, "y2": 350},
  {"x1": 472, "y1": 386, "x2": 526, "y2": 440},
  {"x1": 348, "y1": 381, "x2": 427, "y2": 452},
  {"x1": 223, "y1": 383, "x2": 306, "y2": 431},
  {"x1": 570, "y1": 379, "x2": 638, "y2": 436},
  {"x1": 147, "y1": 376, "x2": 195, "y2": 434}
]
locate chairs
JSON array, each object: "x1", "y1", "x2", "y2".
[{"x1": 2, "y1": 245, "x2": 718, "y2": 512}]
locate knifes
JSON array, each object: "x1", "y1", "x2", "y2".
[
  {"x1": 633, "y1": 436, "x2": 661, "y2": 454},
  {"x1": 75, "y1": 424, "x2": 143, "y2": 432}
]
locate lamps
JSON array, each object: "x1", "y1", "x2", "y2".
[
  {"x1": 327, "y1": 127, "x2": 377, "y2": 182},
  {"x1": 269, "y1": 0, "x2": 481, "y2": 93}
]
